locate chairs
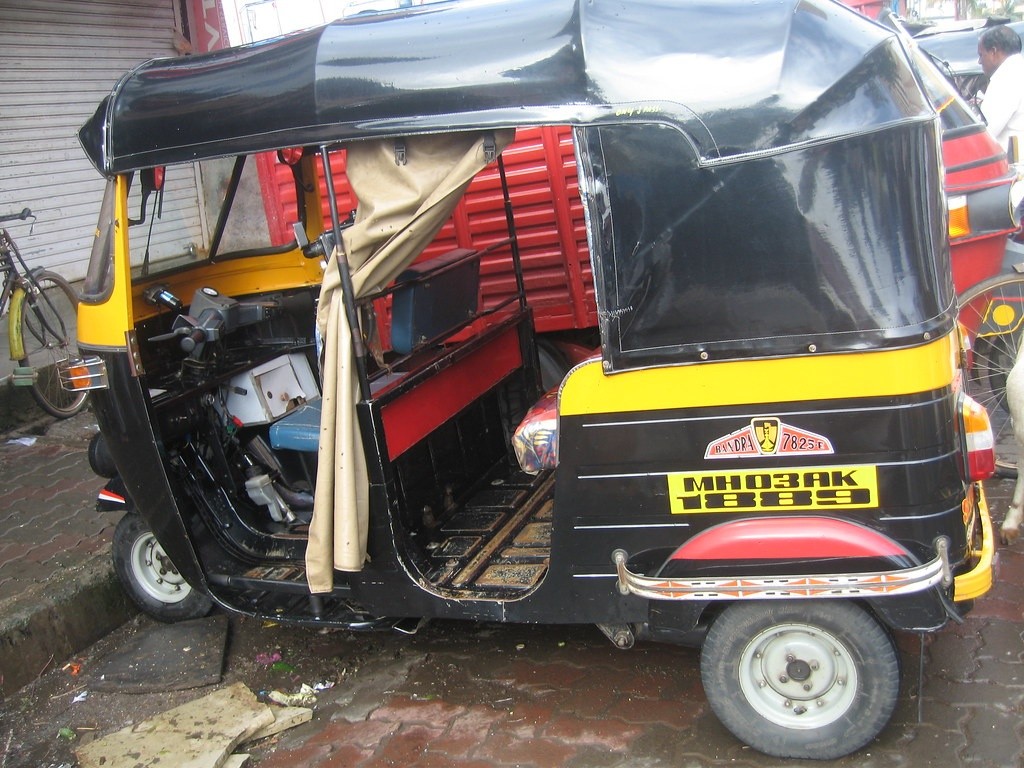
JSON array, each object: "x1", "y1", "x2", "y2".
[{"x1": 269, "y1": 249, "x2": 483, "y2": 494}]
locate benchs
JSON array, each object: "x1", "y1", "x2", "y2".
[{"x1": 511, "y1": 342, "x2": 602, "y2": 477}]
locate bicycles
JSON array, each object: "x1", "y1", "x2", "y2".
[
  {"x1": 955, "y1": 263, "x2": 1024, "y2": 478},
  {"x1": 0, "y1": 207, "x2": 90, "y2": 419}
]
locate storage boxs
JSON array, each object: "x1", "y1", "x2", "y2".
[{"x1": 212, "y1": 349, "x2": 323, "y2": 428}]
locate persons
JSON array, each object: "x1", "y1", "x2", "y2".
[{"x1": 977, "y1": 23, "x2": 1024, "y2": 156}]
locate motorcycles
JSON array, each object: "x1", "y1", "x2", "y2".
[{"x1": 60, "y1": 1, "x2": 1024, "y2": 760}]
